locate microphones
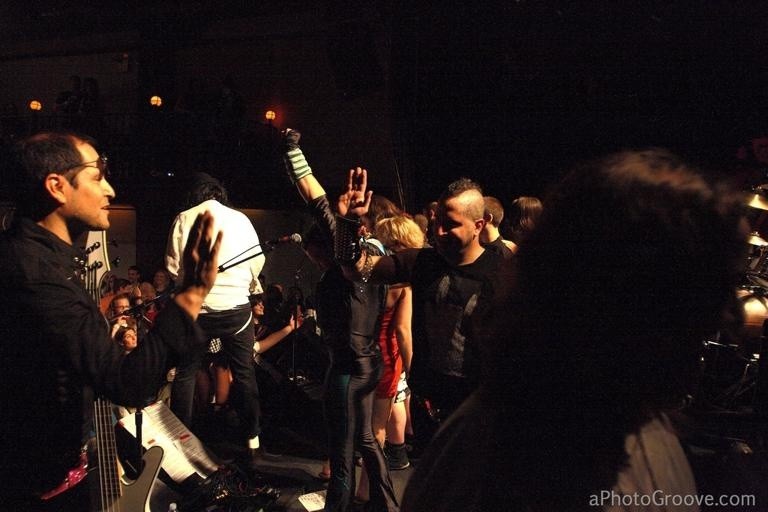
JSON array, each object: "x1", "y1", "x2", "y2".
[{"x1": 267, "y1": 232, "x2": 303, "y2": 247}]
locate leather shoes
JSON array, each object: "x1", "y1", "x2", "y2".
[{"x1": 244, "y1": 435, "x2": 262, "y2": 457}]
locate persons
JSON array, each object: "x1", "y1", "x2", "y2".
[{"x1": 1, "y1": 123, "x2": 768, "y2": 510}]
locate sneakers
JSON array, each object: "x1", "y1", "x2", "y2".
[{"x1": 381, "y1": 447, "x2": 410, "y2": 470}]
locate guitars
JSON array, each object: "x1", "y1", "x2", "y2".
[{"x1": 73, "y1": 230, "x2": 164, "y2": 510}]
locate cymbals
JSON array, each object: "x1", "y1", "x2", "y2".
[
  {"x1": 748, "y1": 235, "x2": 768, "y2": 247},
  {"x1": 737, "y1": 191, "x2": 767, "y2": 211}
]
